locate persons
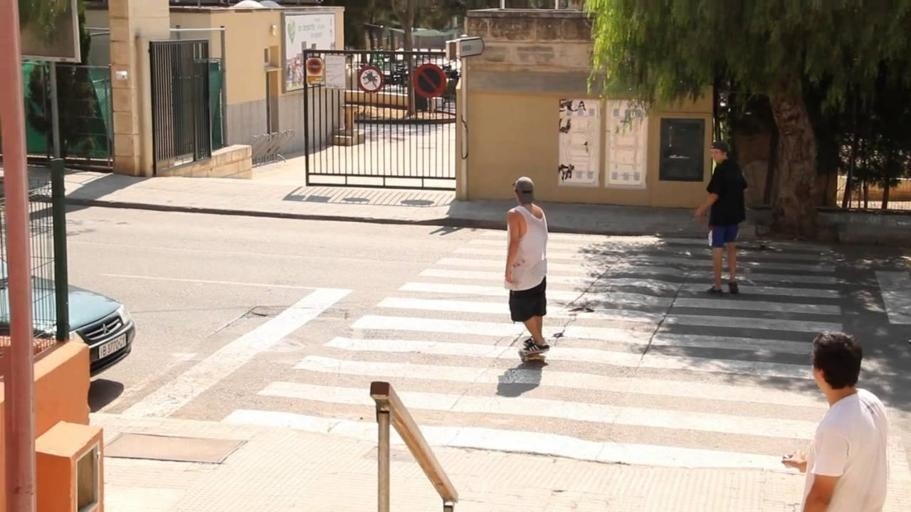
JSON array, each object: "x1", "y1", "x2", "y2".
[
  {"x1": 781, "y1": 330, "x2": 891, "y2": 512},
  {"x1": 693, "y1": 139, "x2": 748, "y2": 295},
  {"x1": 502, "y1": 175, "x2": 549, "y2": 358}
]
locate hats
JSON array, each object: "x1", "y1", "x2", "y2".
[
  {"x1": 514, "y1": 176, "x2": 535, "y2": 204},
  {"x1": 712, "y1": 140, "x2": 731, "y2": 155}
]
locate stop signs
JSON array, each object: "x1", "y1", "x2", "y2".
[{"x1": 306, "y1": 57, "x2": 322, "y2": 76}]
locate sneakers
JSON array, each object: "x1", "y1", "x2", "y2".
[
  {"x1": 520, "y1": 335, "x2": 551, "y2": 357},
  {"x1": 708, "y1": 282, "x2": 739, "y2": 296}
]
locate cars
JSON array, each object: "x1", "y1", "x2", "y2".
[{"x1": 0, "y1": 259, "x2": 136, "y2": 379}]
